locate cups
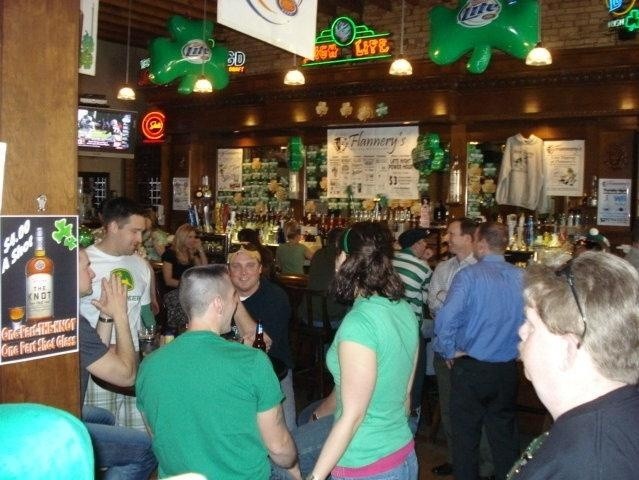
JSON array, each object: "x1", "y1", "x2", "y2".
[
  {"x1": 556, "y1": 261, "x2": 587, "y2": 349},
  {"x1": 229, "y1": 243, "x2": 256, "y2": 253}
]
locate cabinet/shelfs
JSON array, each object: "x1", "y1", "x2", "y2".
[
  {"x1": 526, "y1": 214, "x2": 597, "y2": 247},
  {"x1": 202, "y1": 174, "x2": 212, "y2": 198},
  {"x1": 252, "y1": 319, "x2": 267, "y2": 353},
  {"x1": 436, "y1": 211, "x2": 442, "y2": 222},
  {"x1": 23, "y1": 225, "x2": 56, "y2": 329},
  {"x1": 221, "y1": 200, "x2": 418, "y2": 247},
  {"x1": 196, "y1": 187, "x2": 202, "y2": 198},
  {"x1": 165, "y1": 331, "x2": 176, "y2": 345}
]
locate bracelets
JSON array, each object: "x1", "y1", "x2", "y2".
[
  {"x1": 99, "y1": 317, "x2": 113, "y2": 322},
  {"x1": 312, "y1": 410, "x2": 318, "y2": 421}
]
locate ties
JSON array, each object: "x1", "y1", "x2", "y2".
[{"x1": 78, "y1": 106, "x2": 138, "y2": 160}]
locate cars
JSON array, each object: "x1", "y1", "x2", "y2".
[
  {"x1": 6, "y1": 305, "x2": 26, "y2": 332},
  {"x1": 135, "y1": 324, "x2": 162, "y2": 361}
]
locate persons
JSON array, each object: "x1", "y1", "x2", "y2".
[
  {"x1": 135, "y1": 264, "x2": 302, "y2": 480},
  {"x1": 79, "y1": 198, "x2": 152, "y2": 433},
  {"x1": 160, "y1": 224, "x2": 207, "y2": 343},
  {"x1": 92, "y1": 200, "x2": 159, "y2": 315},
  {"x1": 429, "y1": 218, "x2": 495, "y2": 480},
  {"x1": 276, "y1": 222, "x2": 315, "y2": 275},
  {"x1": 436, "y1": 223, "x2": 528, "y2": 480},
  {"x1": 297, "y1": 226, "x2": 344, "y2": 402},
  {"x1": 392, "y1": 228, "x2": 434, "y2": 431},
  {"x1": 506, "y1": 251, "x2": 639, "y2": 480},
  {"x1": 142, "y1": 214, "x2": 168, "y2": 260},
  {"x1": 271, "y1": 331, "x2": 426, "y2": 480},
  {"x1": 224, "y1": 242, "x2": 297, "y2": 429},
  {"x1": 238, "y1": 229, "x2": 274, "y2": 279},
  {"x1": 310, "y1": 221, "x2": 419, "y2": 480},
  {"x1": 79, "y1": 243, "x2": 158, "y2": 480}
]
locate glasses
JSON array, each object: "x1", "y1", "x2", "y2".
[
  {"x1": 283, "y1": 54, "x2": 305, "y2": 85},
  {"x1": 526, "y1": 0, "x2": 553, "y2": 66},
  {"x1": 389, "y1": 0, "x2": 413, "y2": 76},
  {"x1": 117, "y1": 1, "x2": 136, "y2": 100},
  {"x1": 193, "y1": 0, "x2": 212, "y2": 93}
]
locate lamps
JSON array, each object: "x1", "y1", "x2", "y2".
[{"x1": 285, "y1": 285, "x2": 337, "y2": 400}]
